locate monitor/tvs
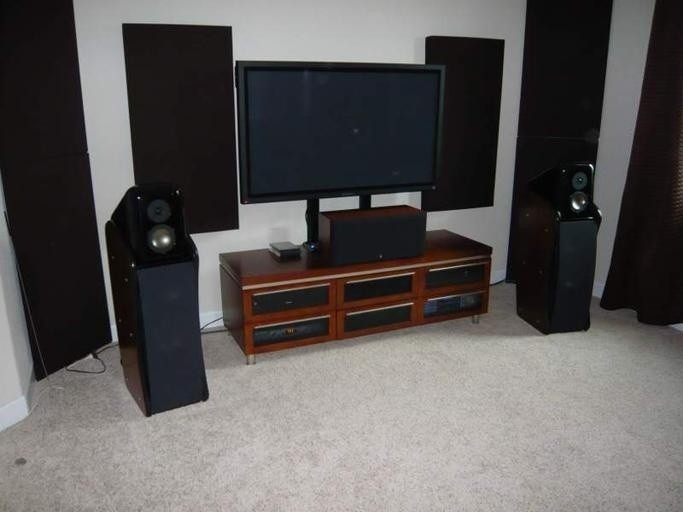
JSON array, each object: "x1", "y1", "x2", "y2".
[{"x1": 235, "y1": 60, "x2": 447, "y2": 252}]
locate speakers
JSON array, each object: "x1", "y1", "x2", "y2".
[
  {"x1": 126, "y1": 184, "x2": 186, "y2": 267},
  {"x1": 555, "y1": 162, "x2": 595, "y2": 220},
  {"x1": 318, "y1": 205, "x2": 427, "y2": 268}
]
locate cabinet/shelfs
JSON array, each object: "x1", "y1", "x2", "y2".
[{"x1": 218, "y1": 229, "x2": 493, "y2": 365}]
